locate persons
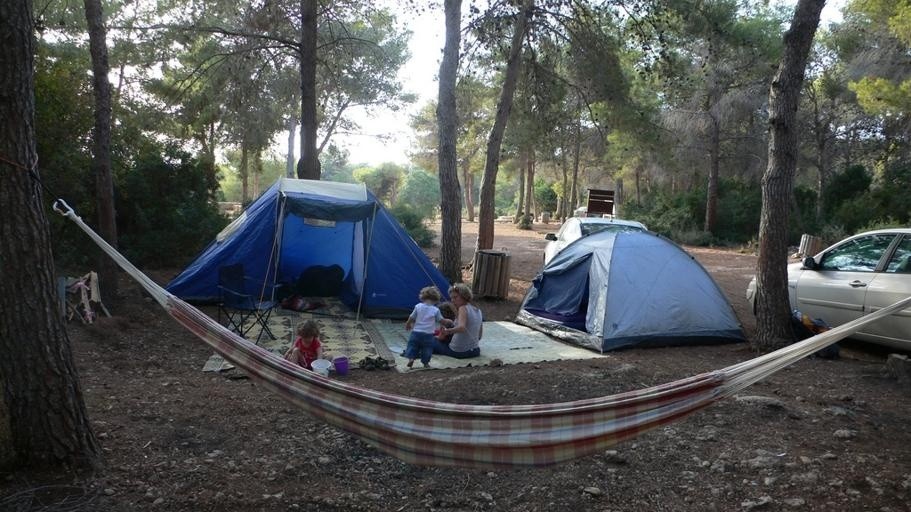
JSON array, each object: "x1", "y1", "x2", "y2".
[
  {"x1": 432, "y1": 300, "x2": 458, "y2": 355},
  {"x1": 402, "y1": 283, "x2": 455, "y2": 368},
  {"x1": 439, "y1": 282, "x2": 485, "y2": 359},
  {"x1": 280, "y1": 318, "x2": 324, "y2": 373}
]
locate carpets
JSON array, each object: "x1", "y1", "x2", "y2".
[
  {"x1": 377, "y1": 318, "x2": 608, "y2": 372},
  {"x1": 204, "y1": 312, "x2": 378, "y2": 372}
]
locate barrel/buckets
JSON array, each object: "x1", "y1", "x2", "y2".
[
  {"x1": 311, "y1": 358, "x2": 331, "y2": 378},
  {"x1": 332, "y1": 357, "x2": 348, "y2": 375}
]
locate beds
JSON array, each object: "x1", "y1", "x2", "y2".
[{"x1": 53, "y1": 196, "x2": 911, "y2": 475}]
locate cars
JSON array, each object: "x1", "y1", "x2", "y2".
[
  {"x1": 746, "y1": 225, "x2": 911, "y2": 356},
  {"x1": 540, "y1": 215, "x2": 648, "y2": 274}
]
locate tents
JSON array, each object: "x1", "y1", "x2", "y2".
[
  {"x1": 513, "y1": 222, "x2": 752, "y2": 355},
  {"x1": 156, "y1": 175, "x2": 454, "y2": 317}
]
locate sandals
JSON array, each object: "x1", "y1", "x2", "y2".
[
  {"x1": 376, "y1": 356, "x2": 388, "y2": 369},
  {"x1": 360, "y1": 357, "x2": 375, "y2": 370}
]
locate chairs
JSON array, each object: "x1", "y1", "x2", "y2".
[{"x1": 217, "y1": 263, "x2": 278, "y2": 345}]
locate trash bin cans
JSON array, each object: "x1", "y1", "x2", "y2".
[
  {"x1": 541, "y1": 212, "x2": 550, "y2": 224},
  {"x1": 798, "y1": 233, "x2": 823, "y2": 258},
  {"x1": 472, "y1": 250, "x2": 512, "y2": 299}
]
